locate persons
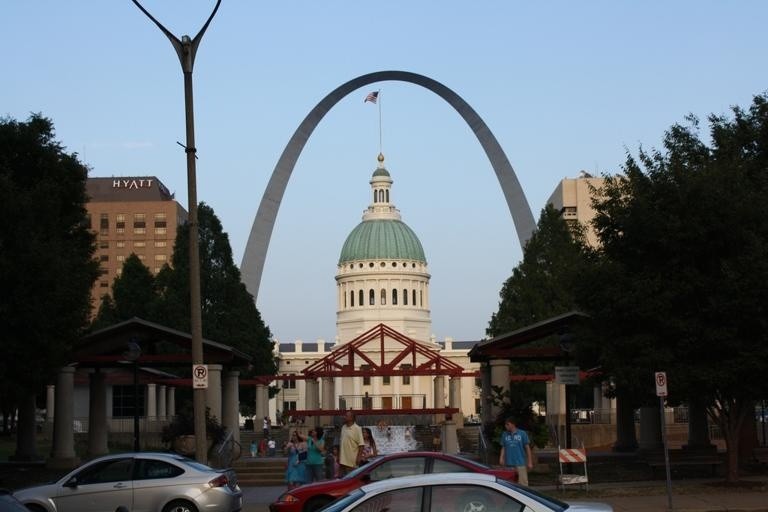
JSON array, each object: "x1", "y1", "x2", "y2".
[
  {"x1": 499, "y1": 416, "x2": 533, "y2": 486},
  {"x1": 263, "y1": 417, "x2": 270, "y2": 438},
  {"x1": 259, "y1": 436, "x2": 268, "y2": 457},
  {"x1": 276, "y1": 409, "x2": 281, "y2": 426},
  {"x1": 284, "y1": 411, "x2": 377, "y2": 490},
  {"x1": 249, "y1": 440, "x2": 258, "y2": 456},
  {"x1": 267, "y1": 437, "x2": 278, "y2": 456}
]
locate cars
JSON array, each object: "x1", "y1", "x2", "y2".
[
  {"x1": 13, "y1": 449, "x2": 244, "y2": 510},
  {"x1": 0, "y1": 489, "x2": 30, "y2": 511},
  {"x1": 312, "y1": 472, "x2": 612, "y2": 512},
  {"x1": 269, "y1": 450, "x2": 520, "y2": 512}
]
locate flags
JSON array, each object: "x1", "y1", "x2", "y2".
[{"x1": 364, "y1": 91, "x2": 378, "y2": 104}]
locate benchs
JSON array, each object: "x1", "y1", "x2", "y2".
[
  {"x1": 639, "y1": 444, "x2": 721, "y2": 479},
  {"x1": 752, "y1": 448, "x2": 768, "y2": 476}
]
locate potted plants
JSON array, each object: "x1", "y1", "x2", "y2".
[{"x1": 161, "y1": 402, "x2": 226, "y2": 458}]
